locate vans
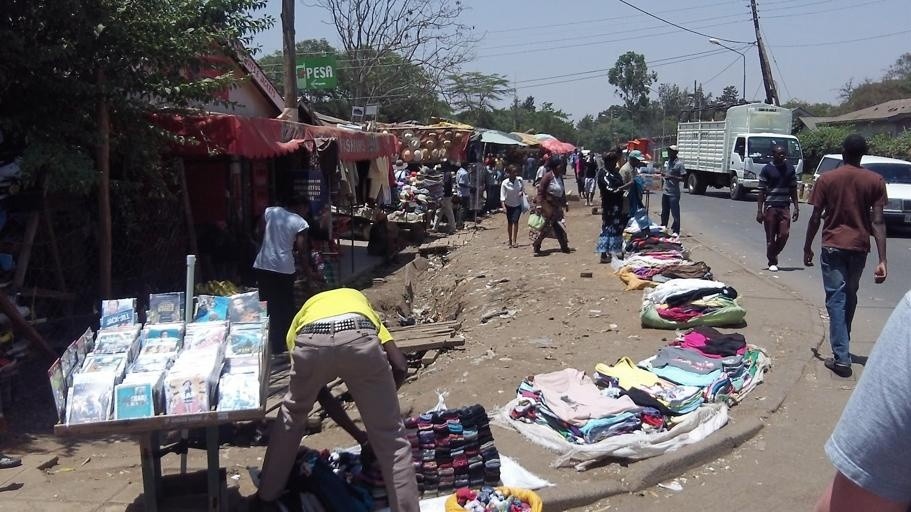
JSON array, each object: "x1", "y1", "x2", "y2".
[{"x1": 813, "y1": 152, "x2": 911, "y2": 231}]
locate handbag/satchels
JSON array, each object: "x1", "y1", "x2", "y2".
[{"x1": 528, "y1": 205, "x2": 546, "y2": 232}]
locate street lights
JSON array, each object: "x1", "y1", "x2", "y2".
[
  {"x1": 600, "y1": 114, "x2": 617, "y2": 128},
  {"x1": 644, "y1": 84, "x2": 666, "y2": 146},
  {"x1": 709, "y1": 38, "x2": 746, "y2": 103}
]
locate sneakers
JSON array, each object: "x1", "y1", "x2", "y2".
[
  {"x1": 824, "y1": 357, "x2": 853, "y2": 378},
  {"x1": 768, "y1": 265, "x2": 778, "y2": 272}
]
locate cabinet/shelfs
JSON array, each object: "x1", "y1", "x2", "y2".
[{"x1": 52, "y1": 347, "x2": 274, "y2": 511}]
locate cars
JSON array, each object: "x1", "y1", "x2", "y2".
[{"x1": 581, "y1": 150, "x2": 591, "y2": 160}]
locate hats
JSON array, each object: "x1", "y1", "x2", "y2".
[
  {"x1": 666, "y1": 145, "x2": 680, "y2": 153},
  {"x1": 628, "y1": 150, "x2": 645, "y2": 162},
  {"x1": 541, "y1": 153, "x2": 552, "y2": 161},
  {"x1": 385, "y1": 128, "x2": 463, "y2": 222}
]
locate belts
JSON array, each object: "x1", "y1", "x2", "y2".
[{"x1": 297, "y1": 315, "x2": 377, "y2": 335}]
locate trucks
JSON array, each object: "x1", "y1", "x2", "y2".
[{"x1": 676, "y1": 102, "x2": 806, "y2": 201}]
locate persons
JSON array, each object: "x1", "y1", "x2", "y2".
[
  {"x1": 256, "y1": 288, "x2": 424, "y2": 512},
  {"x1": 171, "y1": 380, "x2": 207, "y2": 414},
  {"x1": 812, "y1": 287, "x2": 910, "y2": 511},
  {"x1": 428, "y1": 146, "x2": 643, "y2": 264},
  {"x1": 757, "y1": 143, "x2": 799, "y2": 271},
  {"x1": 368, "y1": 214, "x2": 402, "y2": 267},
  {"x1": 660, "y1": 145, "x2": 687, "y2": 238},
  {"x1": 803, "y1": 133, "x2": 886, "y2": 373},
  {"x1": 108, "y1": 300, "x2": 120, "y2": 314},
  {"x1": 233, "y1": 299, "x2": 258, "y2": 321},
  {"x1": 252, "y1": 195, "x2": 323, "y2": 360},
  {"x1": 159, "y1": 332, "x2": 169, "y2": 338}
]
